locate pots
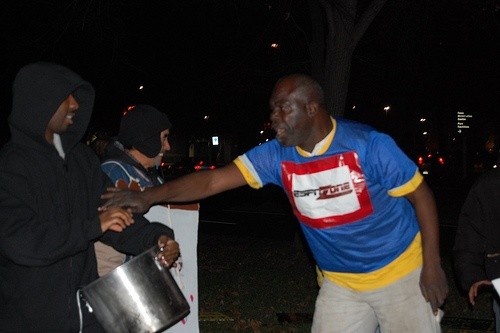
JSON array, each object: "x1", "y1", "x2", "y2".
[{"x1": 76, "y1": 246, "x2": 190, "y2": 333}]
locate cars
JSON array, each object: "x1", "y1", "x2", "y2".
[{"x1": 160, "y1": 152, "x2": 216, "y2": 182}]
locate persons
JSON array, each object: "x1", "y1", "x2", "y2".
[
  {"x1": 0, "y1": 62, "x2": 180, "y2": 333},
  {"x1": 97, "y1": 75, "x2": 450, "y2": 333},
  {"x1": 452, "y1": 170, "x2": 500, "y2": 333},
  {"x1": 94, "y1": 104, "x2": 172, "y2": 279},
  {"x1": 92, "y1": 133, "x2": 500, "y2": 174}
]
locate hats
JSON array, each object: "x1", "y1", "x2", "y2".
[{"x1": 119, "y1": 104, "x2": 172, "y2": 158}]
